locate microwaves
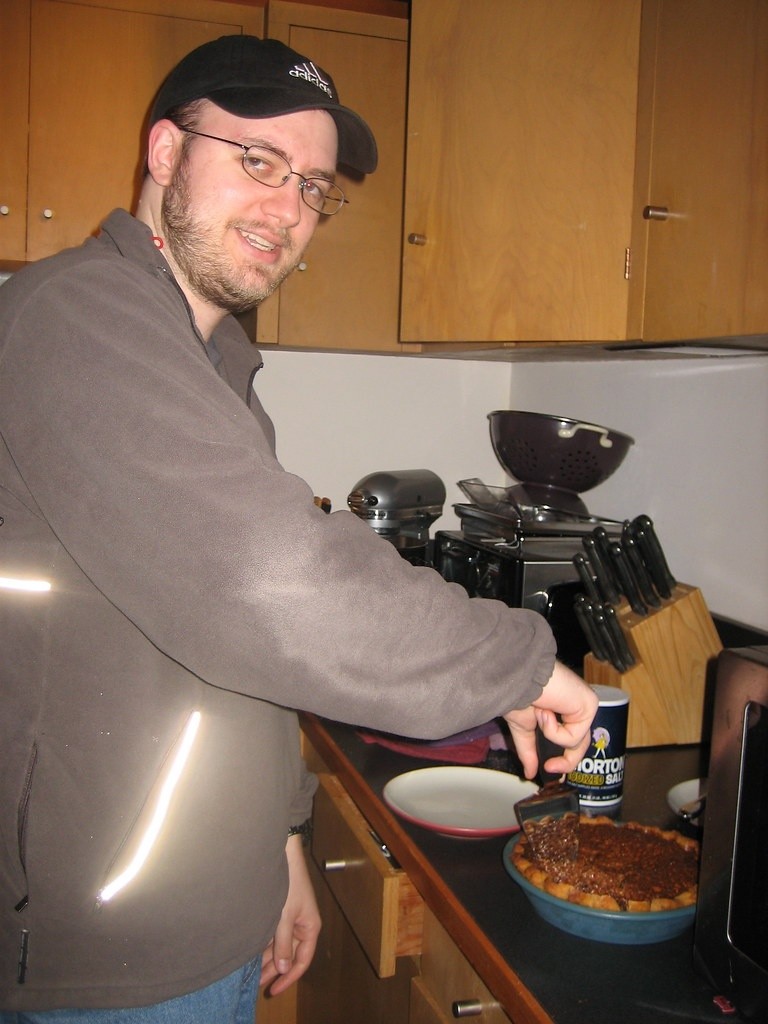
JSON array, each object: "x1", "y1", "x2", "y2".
[{"x1": 435, "y1": 528, "x2": 595, "y2": 678}]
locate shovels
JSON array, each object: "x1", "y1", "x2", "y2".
[{"x1": 517, "y1": 711, "x2": 582, "y2": 877}]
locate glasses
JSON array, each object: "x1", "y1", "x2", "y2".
[{"x1": 176, "y1": 127, "x2": 350, "y2": 217}]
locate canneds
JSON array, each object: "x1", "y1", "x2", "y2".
[{"x1": 565, "y1": 684, "x2": 629, "y2": 807}]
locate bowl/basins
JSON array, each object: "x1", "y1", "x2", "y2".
[{"x1": 502, "y1": 813, "x2": 697, "y2": 945}]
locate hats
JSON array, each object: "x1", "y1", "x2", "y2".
[{"x1": 150, "y1": 34, "x2": 376, "y2": 177}]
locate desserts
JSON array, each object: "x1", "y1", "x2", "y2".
[{"x1": 503, "y1": 812, "x2": 702, "y2": 943}]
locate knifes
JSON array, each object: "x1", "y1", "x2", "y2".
[{"x1": 572, "y1": 515, "x2": 678, "y2": 674}]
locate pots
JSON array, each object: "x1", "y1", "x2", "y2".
[{"x1": 487, "y1": 410, "x2": 636, "y2": 494}]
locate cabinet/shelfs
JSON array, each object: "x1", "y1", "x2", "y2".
[
  {"x1": 0, "y1": 0, "x2": 264, "y2": 274},
  {"x1": 637, "y1": 0, "x2": 768, "y2": 355},
  {"x1": 299, "y1": 729, "x2": 507, "y2": 1024},
  {"x1": 255, "y1": 1, "x2": 423, "y2": 358},
  {"x1": 400, "y1": 0, "x2": 640, "y2": 361}
]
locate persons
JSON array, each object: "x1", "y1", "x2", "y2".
[{"x1": 1, "y1": 33, "x2": 599, "y2": 1023}]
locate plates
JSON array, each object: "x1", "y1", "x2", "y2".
[{"x1": 383, "y1": 766, "x2": 539, "y2": 841}]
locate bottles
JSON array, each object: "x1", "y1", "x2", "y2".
[{"x1": 564, "y1": 683, "x2": 629, "y2": 808}]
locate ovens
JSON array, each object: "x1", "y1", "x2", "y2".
[{"x1": 694, "y1": 643, "x2": 767, "y2": 1024}]
